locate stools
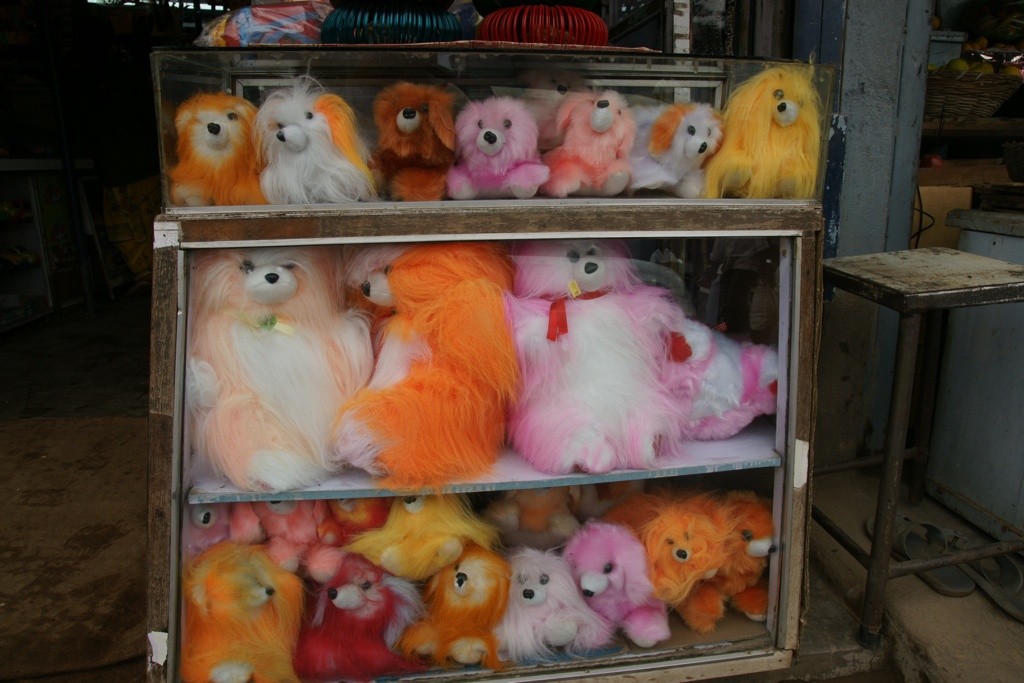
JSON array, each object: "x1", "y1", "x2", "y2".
[{"x1": 813, "y1": 245, "x2": 1024, "y2": 648}]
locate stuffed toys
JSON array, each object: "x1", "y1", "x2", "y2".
[{"x1": 170, "y1": 62, "x2": 826, "y2": 683}]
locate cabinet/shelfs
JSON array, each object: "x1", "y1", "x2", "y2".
[
  {"x1": 0, "y1": 178, "x2": 50, "y2": 328},
  {"x1": 149, "y1": 204, "x2": 827, "y2": 683}
]
